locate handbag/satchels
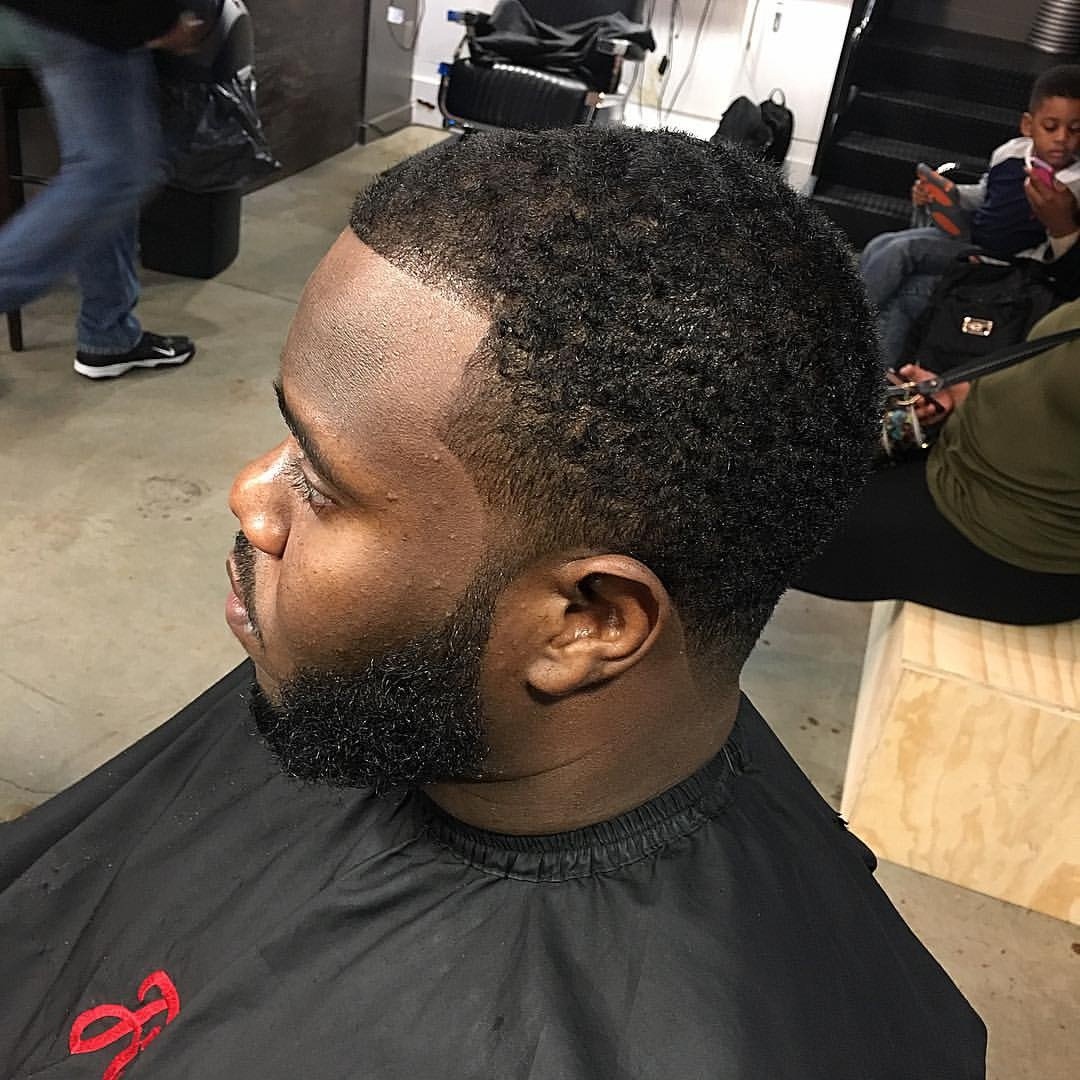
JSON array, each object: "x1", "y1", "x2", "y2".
[
  {"x1": 152, "y1": 51, "x2": 282, "y2": 193},
  {"x1": 902, "y1": 249, "x2": 1060, "y2": 375},
  {"x1": 710, "y1": 89, "x2": 795, "y2": 173}
]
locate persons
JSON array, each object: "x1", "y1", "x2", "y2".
[
  {"x1": 0, "y1": 118, "x2": 989, "y2": 1080},
  {"x1": 0, "y1": 0, "x2": 203, "y2": 380},
  {"x1": 788, "y1": 64, "x2": 1080, "y2": 627}
]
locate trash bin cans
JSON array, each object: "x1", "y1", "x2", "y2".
[{"x1": 139, "y1": 75, "x2": 255, "y2": 278}]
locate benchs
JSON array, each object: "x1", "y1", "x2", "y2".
[{"x1": 840, "y1": 600, "x2": 1078, "y2": 926}]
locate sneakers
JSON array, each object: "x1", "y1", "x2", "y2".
[{"x1": 73, "y1": 331, "x2": 197, "y2": 381}]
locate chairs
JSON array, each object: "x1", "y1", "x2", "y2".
[{"x1": 439, "y1": 1, "x2": 658, "y2": 132}]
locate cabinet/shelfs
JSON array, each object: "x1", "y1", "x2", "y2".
[{"x1": 803, "y1": 0, "x2": 1080, "y2": 252}]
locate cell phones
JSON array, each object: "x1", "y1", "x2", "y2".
[
  {"x1": 1029, "y1": 156, "x2": 1054, "y2": 195},
  {"x1": 884, "y1": 366, "x2": 945, "y2": 411},
  {"x1": 916, "y1": 163, "x2": 967, "y2": 241}
]
